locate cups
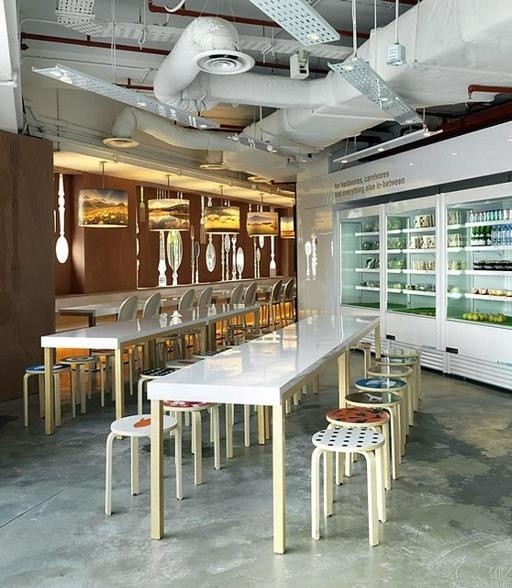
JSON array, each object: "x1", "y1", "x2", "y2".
[
  {"x1": 387, "y1": 281, "x2": 406, "y2": 289},
  {"x1": 405, "y1": 211, "x2": 465, "y2": 290}
]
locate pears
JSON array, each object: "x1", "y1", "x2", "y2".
[{"x1": 462, "y1": 312, "x2": 507, "y2": 322}]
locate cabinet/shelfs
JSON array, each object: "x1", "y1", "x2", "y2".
[{"x1": 335, "y1": 179, "x2": 512, "y2": 393}]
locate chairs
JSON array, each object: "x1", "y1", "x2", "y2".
[{"x1": 115, "y1": 277, "x2": 295, "y2": 361}]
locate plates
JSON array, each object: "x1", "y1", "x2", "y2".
[{"x1": 389, "y1": 219, "x2": 405, "y2": 268}]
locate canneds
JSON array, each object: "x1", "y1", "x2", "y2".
[{"x1": 469, "y1": 209, "x2": 512, "y2": 222}]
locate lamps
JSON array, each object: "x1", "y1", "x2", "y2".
[
  {"x1": 77, "y1": 160, "x2": 296, "y2": 238},
  {"x1": 386, "y1": 0, "x2": 408, "y2": 68}
]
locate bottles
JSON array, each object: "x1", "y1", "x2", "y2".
[
  {"x1": 468, "y1": 209, "x2": 512, "y2": 246},
  {"x1": 362, "y1": 240, "x2": 378, "y2": 251}
]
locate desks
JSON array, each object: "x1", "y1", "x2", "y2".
[{"x1": 53, "y1": 274, "x2": 297, "y2": 369}]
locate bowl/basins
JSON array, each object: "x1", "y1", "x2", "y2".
[{"x1": 474, "y1": 260, "x2": 512, "y2": 271}]
